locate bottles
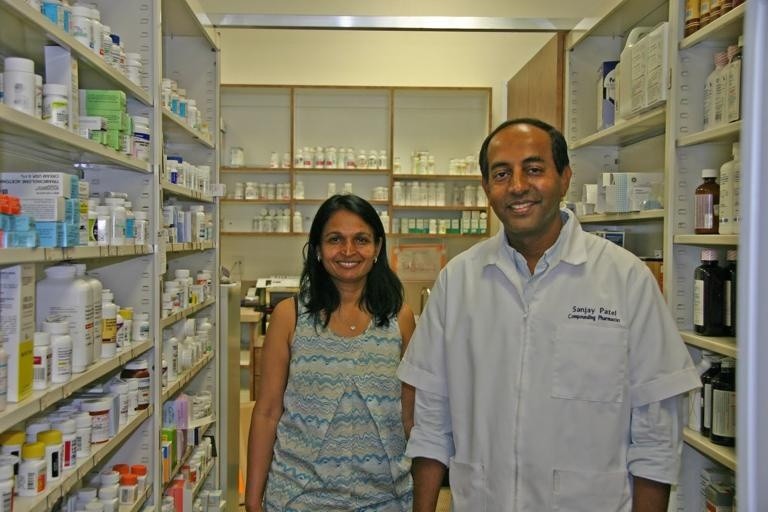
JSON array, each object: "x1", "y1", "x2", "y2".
[
  {"x1": 1, "y1": 0, "x2": 153, "y2": 512},
  {"x1": 161, "y1": 77, "x2": 219, "y2": 510},
  {"x1": 221, "y1": 144, "x2": 489, "y2": 235},
  {"x1": 680, "y1": 2, "x2": 745, "y2": 510}
]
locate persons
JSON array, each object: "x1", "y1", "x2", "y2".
[
  {"x1": 242, "y1": 193, "x2": 416, "y2": 510},
  {"x1": 393, "y1": 119, "x2": 703, "y2": 511}
]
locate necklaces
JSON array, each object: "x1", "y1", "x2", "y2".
[{"x1": 331, "y1": 305, "x2": 367, "y2": 335}]
